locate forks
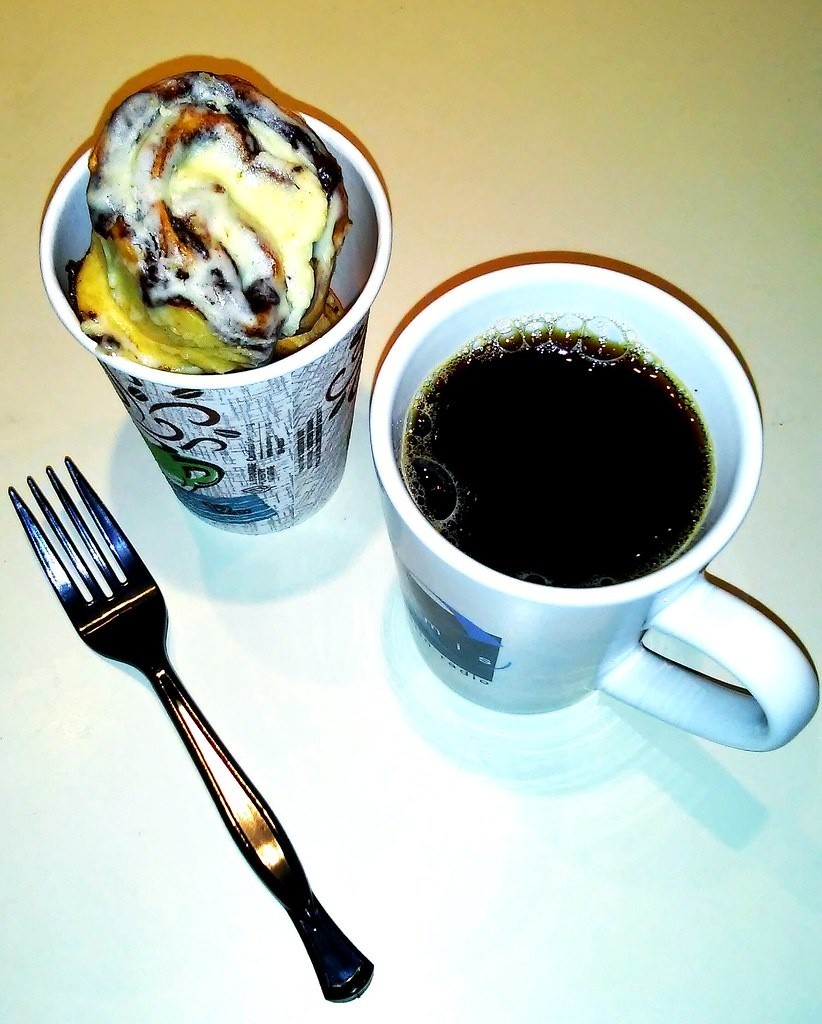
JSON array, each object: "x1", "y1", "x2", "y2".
[{"x1": 9, "y1": 455, "x2": 378, "y2": 1007}]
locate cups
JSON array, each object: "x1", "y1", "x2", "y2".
[
  {"x1": 368, "y1": 258, "x2": 818, "y2": 751},
  {"x1": 37, "y1": 102, "x2": 394, "y2": 534}
]
